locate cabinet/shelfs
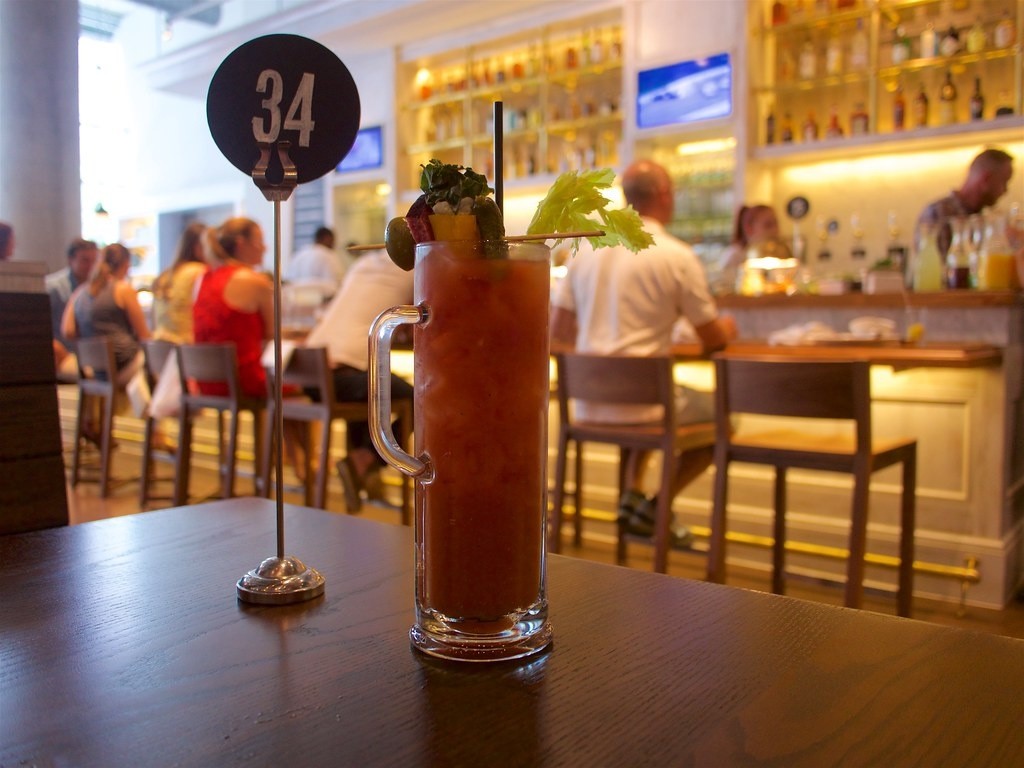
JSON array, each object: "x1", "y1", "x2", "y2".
[
  {"x1": 399, "y1": 10, "x2": 622, "y2": 189},
  {"x1": 748, "y1": 0, "x2": 1024, "y2": 146}
]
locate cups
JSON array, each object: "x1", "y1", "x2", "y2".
[
  {"x1": 905, "y1": 306, "x2": 927, "y2": 347},
  {"x1": 366, "y1": 239, "x2": 552, "y2": 664}
]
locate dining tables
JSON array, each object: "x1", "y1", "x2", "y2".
[{"x1": 0, "y1": 497, "x2": 1024, "y2": 768}]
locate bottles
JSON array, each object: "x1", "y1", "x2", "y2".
[
  {"x1": 763, "y1": 1, "x2": 1017, "y2": 144},
  {"x1": 410, "y1": 21, "x2": 623, "y2": 179},
  {"x1": 777, "y1": 202, "x2": 1024, "y2": 293}
]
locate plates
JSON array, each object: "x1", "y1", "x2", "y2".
[{"x1": 819, "y1": 334, "x2": 902, "y2": 346}]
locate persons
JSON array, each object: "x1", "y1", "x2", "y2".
[
  {"x1": 153, "y1": 218, "x2": 321, "y2": 498},
  {"x1": 913, "y1": 150, "x2": 1014, "y2": 283},
  {"x1": 46, "y1": 239, "x2": 177, "y2": 455},
  {"x1": 549, "y1": 161, "x2": 739, "y2": 549},
  {"x1": 302, "y1": 250, "x2": 414, "y2": 516},
  {"x1": 720, "y1": 205, "x2": 790, "y2": 277},
  {"x1": 289, "y1": 228, "x2": 340, "y2": 285},
  {"x1": 0, "y1": 223, "x2": 15, "y2": 260}
]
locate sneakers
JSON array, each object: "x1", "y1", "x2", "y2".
[
  {"x1": 627, "y1": 498, "x2": 692, "y2": 546},
  {"x1": 616, "y1": 492, "x2": 648, "y2": 528},
  {"x1": 337, "y1": 456, "x2": 364, "y2": 518}
]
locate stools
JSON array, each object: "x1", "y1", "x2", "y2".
[{"x1": 72, "y1": 340, "x2": 915, "y2": 618}]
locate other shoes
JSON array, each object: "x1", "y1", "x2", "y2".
[{"x1": 78, "y1": 432, "x2": 119, "y2": 452}]
